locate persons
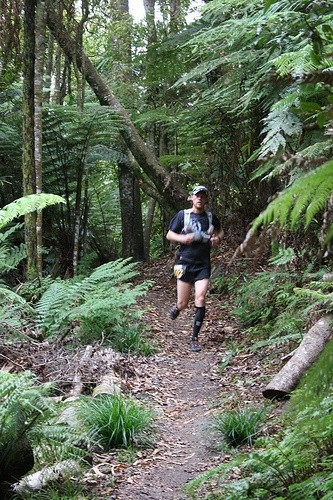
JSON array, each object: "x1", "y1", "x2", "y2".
[{"x1": 165, "y1": 186, "x2": 225, "y2": 352}]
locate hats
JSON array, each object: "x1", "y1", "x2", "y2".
[{"x1": 192, "y1": 186, "x2": 208, "y2": 198}]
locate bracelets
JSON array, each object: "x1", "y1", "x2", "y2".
[{"x1": 216, "y1": 235, "x2": 220, "y2": 243}]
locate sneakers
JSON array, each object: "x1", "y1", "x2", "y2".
[
  {"x1": 189, "y1": 337, "x2": 202, "y2": 352},
  {"x1": 169, "y1": 303, "x2": 180, "y2": 321}
]
